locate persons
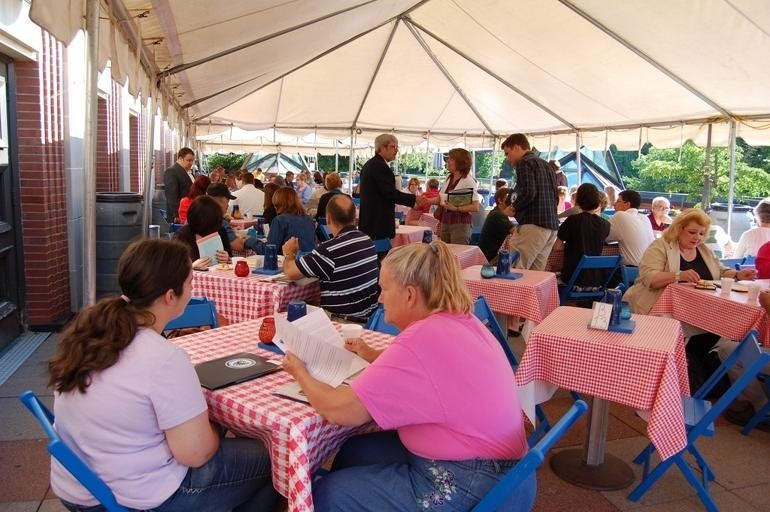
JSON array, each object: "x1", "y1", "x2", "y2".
[
  {"x1": 702, "y1": 225, "x2": 733, "y2": 258},
  {"x1": 605, "y1": 190, "x2": 654, "y2": 287},
  {"x1": 283, "y1": 239, "x2": 530, "y2": 512},
  {"x1": 163, "y1": 133, "x2": 617, "y2": 325},
  {"x1": 42, "y1": 239, "x2": 281, "y2": 510},
  {"x1": 647, "y1": 196, "x2": 672, "y2": 232},
  {"x1": 718, "y1": 290, "x2": 770, "y2": 432},
  {"x1": 621, "y1": 210, "x2": 758, "y2": 402},
  {"x1": 733, "y1": 201, "x2": 770, "y2": 258}
]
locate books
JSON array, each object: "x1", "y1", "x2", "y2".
[{"x1": 193, "y1": 351, "x2": 280, "y2": 390}]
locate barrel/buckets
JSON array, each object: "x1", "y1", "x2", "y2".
[
  {"x1": 96, "y1": 192, "x2": 144, "y2": 300},
  {"x1": 152, "y1": 183, "x2": 171, "y2": 233}
]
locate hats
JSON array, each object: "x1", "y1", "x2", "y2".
[{"x1": 207, "y1": 183, "x2": 236, "y2": 199}]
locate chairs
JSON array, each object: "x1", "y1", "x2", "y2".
[{"x1": 19, "y1": 390, "x2": 130, "y2": 512}]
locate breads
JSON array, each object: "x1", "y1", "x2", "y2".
[
  {"x1": 218, "y1": 264, "x2": 228, "y2": 268},
  {"x1": 697, "y1": 280, "x2": 713, "y2": 285}
]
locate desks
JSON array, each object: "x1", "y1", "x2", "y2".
[{"x1": 167, "y1": 204, "x2": 770, "y2": 512}]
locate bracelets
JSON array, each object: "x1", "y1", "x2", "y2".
[
  {"x1": 735, "y1": 271, "x2": 740, "y2": 280},
  {"x1": 675, "y1": 270, "x2": 681, "y2": 281}
]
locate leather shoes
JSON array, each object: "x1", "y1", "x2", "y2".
[{"x1": 726, "y1": 406, "x2": 770, "y2": 432}]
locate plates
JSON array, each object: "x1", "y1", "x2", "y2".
[
  {"x1": 713, "y1": 281, "x2": 748, "y2": 293},
  {"x1": 215, "y1": 264, "x2": 232, "y2": 270}
]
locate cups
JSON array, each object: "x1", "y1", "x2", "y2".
[
  {"x1": 395, "y1": 218, "x2": 401, "y2": 229},
  {"x1": 233, "y1": 204, "x2": 255, "y2": 220},
  {"x1": 721, "y1": 277, "x2": 732, "y2": 294},
  {"x1": 232, "y1": 257, "x2": 244, "y2": 269},
  {"x1": 422, "y1": 229, "x2": 432, "y2": 243},
  {"x1": 285, "y1": 300, "x2": 307, "y2": 322},
  {"x1": 497, "y1": 250, "x2": 511, "y2": 275},
  {"x1": 748, "y1": 282, "x2": 760, "y2": 301},
  {"x1": 263, "y1": 244, "x2": 278, "y2": 271},
  {"x1": 605, "y1": 288, "x2": 622, "y2": 326},
  {"x1": 342, "y1": 321, "x2": 364, "y2": 341}
]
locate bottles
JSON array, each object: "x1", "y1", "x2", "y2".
[
  {"x1": 258, "y1": 317, "x2": 276, "y2": 345},
  {"x1": 618, "y1": 301, "x2": 632, "y2": 320},
  {"x1": 235, "y1": 261, "x2": 249, "y2": 277},
  {"x1": 247, "y1": 229, "x2": 258, "y2": 239},
  {"x1": 480, "y1": 263, "x2": 496, "y2": 278}
]
